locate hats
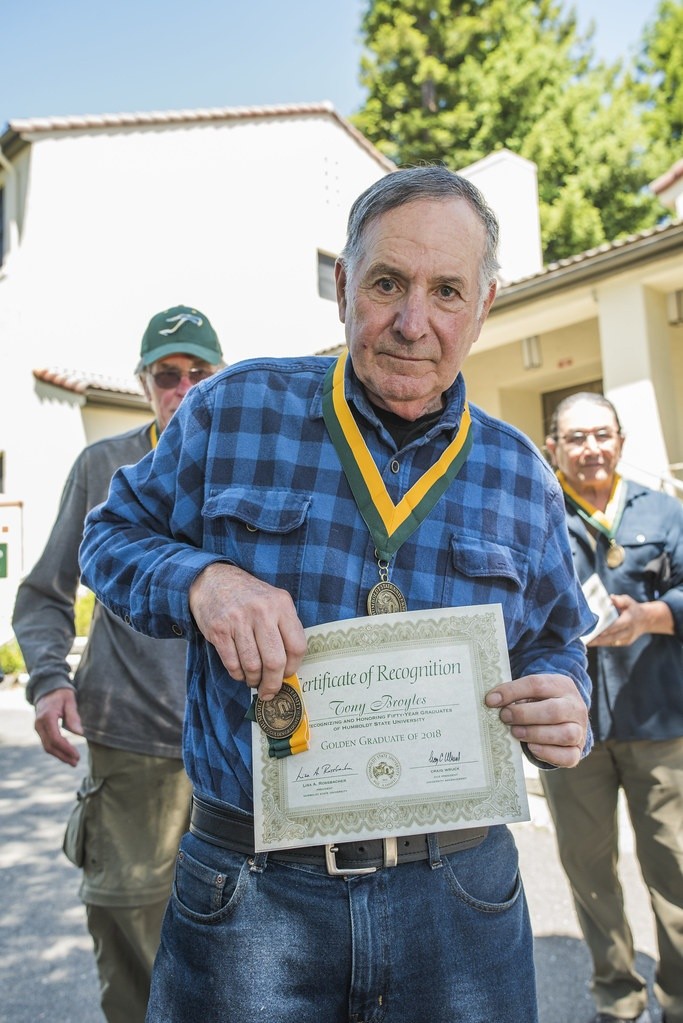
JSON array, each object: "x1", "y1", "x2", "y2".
[{"x1": 140, "y1": 304, "x2": 223, "y2": 366}]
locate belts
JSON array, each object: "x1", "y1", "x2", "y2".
[{"x1": 189, "y1": 794, "x2": 492, "y2": 875}]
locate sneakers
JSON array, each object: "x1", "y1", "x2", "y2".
[
  {"x1": 587, "y1": 1008, "x2": 651, "y2": 1023},
  {"x1": 657, "y1": 1009, "x2": 665, "y2": 1022}
]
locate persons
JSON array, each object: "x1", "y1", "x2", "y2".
[
  {"x1": 538, "y1": 392, "x2": 683, "y2": 1022},
  {"x1": 11, "y1": 305, "x2": 229, "y2": 1023},
  {"x1": 75, "y1": 165, "x2": 593, "y2": 1023}
]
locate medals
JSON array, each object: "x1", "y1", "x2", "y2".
[
  {"x1": 256, "y1": 682, "x2": 303, "y2": 738},
  {"x1": 606, "y1": 545, "x2": 625, "y2": 568},
  {"x1": 367, "y1": 583, "x2": 408, "y2": 617}
]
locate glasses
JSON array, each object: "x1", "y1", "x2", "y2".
[
  {"x1": 144, "y1": 367, "x2": 220, "y2": 390},
  {"x1": 555, "y1": 430, "x2": 615, "y2": 445}
]
